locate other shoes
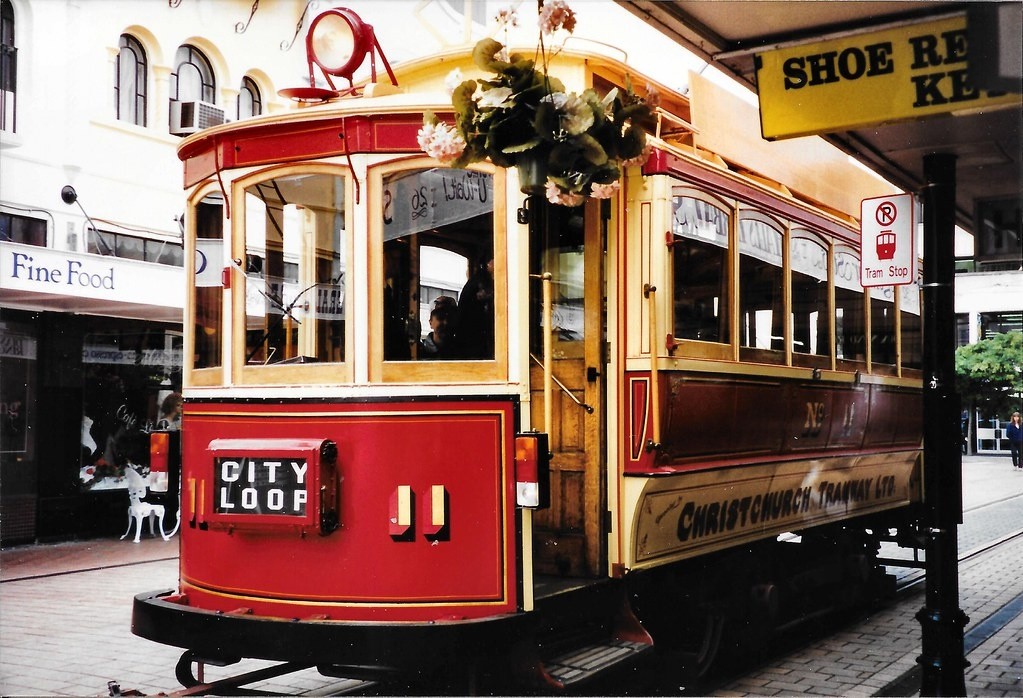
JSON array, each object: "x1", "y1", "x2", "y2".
[
  {"x1": 1013, "y1": 466, "x2": 1017, "y2": 470},
  {"x1": 1019, "y1": 468, "x2": 1022, "y2": 471}
]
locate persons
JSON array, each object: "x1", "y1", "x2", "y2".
[
  {"x1": 1005, "y1": 412, "x2": 1023, "y2": 468},
  {"x1": 157, "y1": 392, "x2": 182, "y2": 423},
  {"x1": 422, "y1": 296, "x2": 456, "y2": 360},
  {"x1": 442, "y1": 253, "x2": 495, "y2": 360}
]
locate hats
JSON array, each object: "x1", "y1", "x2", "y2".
[{"x1": 429, "y1": 295, "x2": 457, "y2": 320}]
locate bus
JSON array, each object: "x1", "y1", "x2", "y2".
[{"x1": 130, "y1": 6, "x2": 928, "y2": 687}]
